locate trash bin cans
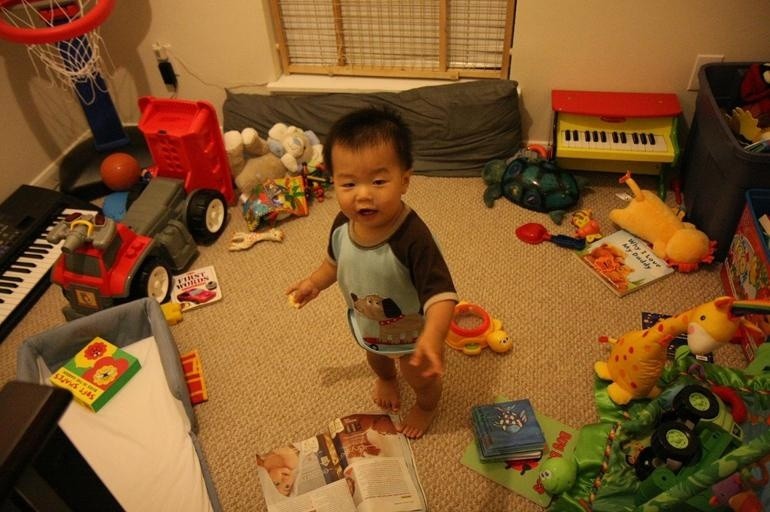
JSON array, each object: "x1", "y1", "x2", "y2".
[{"x1": 680, "y1": 61, "x2": 770, "y2": 261}]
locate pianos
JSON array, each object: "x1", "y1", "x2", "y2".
[{"x1": 0, "y1": 183, "x2": 102, "y2": 342}]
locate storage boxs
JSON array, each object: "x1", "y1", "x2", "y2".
[{"x1": 680, "y1": 59, "x2": 769, "y2": 363}]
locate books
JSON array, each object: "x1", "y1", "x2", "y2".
[
  {"x1": 470, "y1": 397, "x2": 546, "y2": 462},
  {"x1": 254, "y1": 412, "x2": 430, "y2": 512},
  {"x1": 458, "y1": 394, "x2": 580, "y2": 507},
  {"x1": 572, "y1": 230, "x2": 675, "y2": 297},
  {"x1": 169, "y1": 265, "x2": 223, "y2": 313}
]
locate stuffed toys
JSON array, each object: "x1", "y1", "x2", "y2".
[
  {"x1": 594, "y1": 297, "x2": 764, "y2": 406},
  {"x1": 220, "y1": 121, "x2": 326, "y2": 203},
  {"x1": 480, "y1": 62, "x2": 769, "y2": 512},
  {"x1": 609, "y1": 169, "x2": 718, "y2": 272},
  {"x1": 484, "y1": 150, "x2": 588, "y2": 226}
]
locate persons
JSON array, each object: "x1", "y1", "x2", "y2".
[
  {"x1": 255, "y1": 442, "x2": 300, "y2": 497},
  {"x1": 283, "y1": 104, "x2": 459, "y2": 440}
]
